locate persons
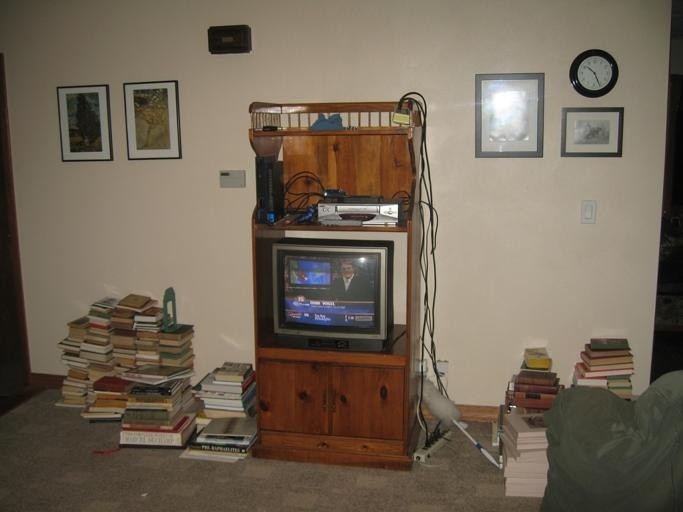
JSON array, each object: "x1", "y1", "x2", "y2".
[{"x1": 330, "y1": 260, "x2": 371, "y2": 296}]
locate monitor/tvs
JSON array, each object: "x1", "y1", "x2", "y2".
[{"x1": 272, "y1": 242, "x2": 390, "y2": 340}]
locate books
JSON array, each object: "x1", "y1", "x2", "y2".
[
  {"x1": 492, "y1": 347, "x2": 565, "y2": 497},
  {"x1": 54, "y1": 294, "x2": 257, "y2": 463},
  {"x1": 571, "y1": 339, "x2": 634, "y2": 401}
]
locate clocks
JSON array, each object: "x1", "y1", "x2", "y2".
[{"x1": 570, "y1": 47, "x2": 620, "y2": 98}]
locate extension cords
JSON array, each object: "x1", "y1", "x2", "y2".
[
  {"x1": 392, "y1": 109, "x2": 410, "y2": 125},
  {"x1": 413, "y1": 429, "x2": 452, "y2": 463}
]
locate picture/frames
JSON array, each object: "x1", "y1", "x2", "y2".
[
  {"x1": 560, "y1": 108, "x2": 625, "y2": 160},
  {"x1": 474, "y1": 71, "x2": 546, "y2": 159},
  {"x1": 56, "y1": 78, "x2": 184, "y2": 163}
]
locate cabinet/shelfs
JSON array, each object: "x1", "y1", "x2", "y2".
[{"x1": 246, "y1": 99, "x2": 428, "y2": 471}]
